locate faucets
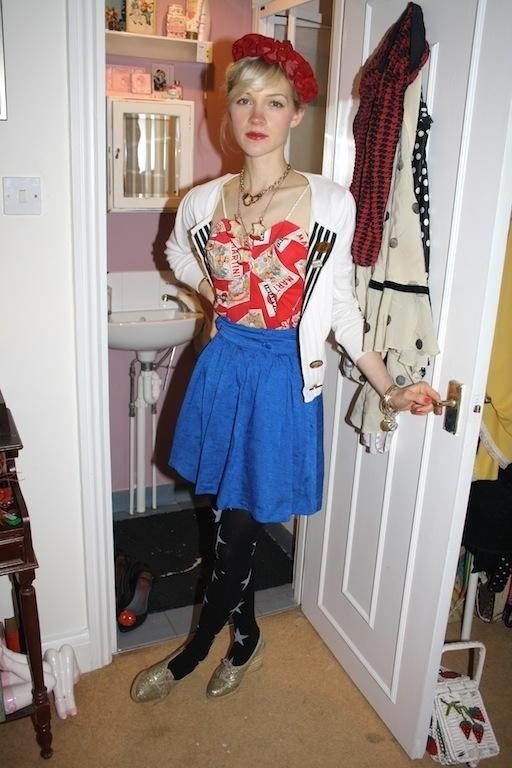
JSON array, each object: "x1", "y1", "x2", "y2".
[{"x1": 161, "y1": 294, "x2": 192, "y2": 312}]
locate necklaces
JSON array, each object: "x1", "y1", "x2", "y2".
[{"x1": 239, "y1": 163, "x2": 291, "y2": 206}]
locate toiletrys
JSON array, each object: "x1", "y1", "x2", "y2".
[
  {"x1": 168, "y1": 85, "x2": 179, "y2": 100},
  {"x1": 173, "y1": 80, "x2": 183, "y2": 99}
]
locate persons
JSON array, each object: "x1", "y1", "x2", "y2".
[
  {"x1": 154, "y1": 69, "x2": 167, "y2": 91},
  {"x1": 130, "y1": 34, "x2": 444, "y2": 704}
]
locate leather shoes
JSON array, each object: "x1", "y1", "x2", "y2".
[{"x1": 118, "y1": 567, "x2": 155, "y2": 633}]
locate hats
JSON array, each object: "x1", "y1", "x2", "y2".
[{"x1": 231, "y1": 34, "x2": 320, "y2": 103}]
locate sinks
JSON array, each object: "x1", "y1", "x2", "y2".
[{"x1": 109, "y1": 272, "x2": 205, "y2": 351}]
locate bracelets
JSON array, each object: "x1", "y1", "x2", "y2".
[{"x1": 380, "y1": 385, "x2": 401, "y2": 432}]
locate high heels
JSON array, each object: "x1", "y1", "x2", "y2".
[
  {"x1": 131, "y1": 645, "x2": 200, "y2": 705},
  {"x1": 207, "y1": 626, "x2": 266, "y2": 699}
]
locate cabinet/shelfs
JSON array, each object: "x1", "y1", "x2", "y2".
[
  {"x1": 106, "y1": 97, "x2": 195, "y2": 215},
  {"x1": 0, "y1": 391, "x2": 53, "y2": 759}
]
